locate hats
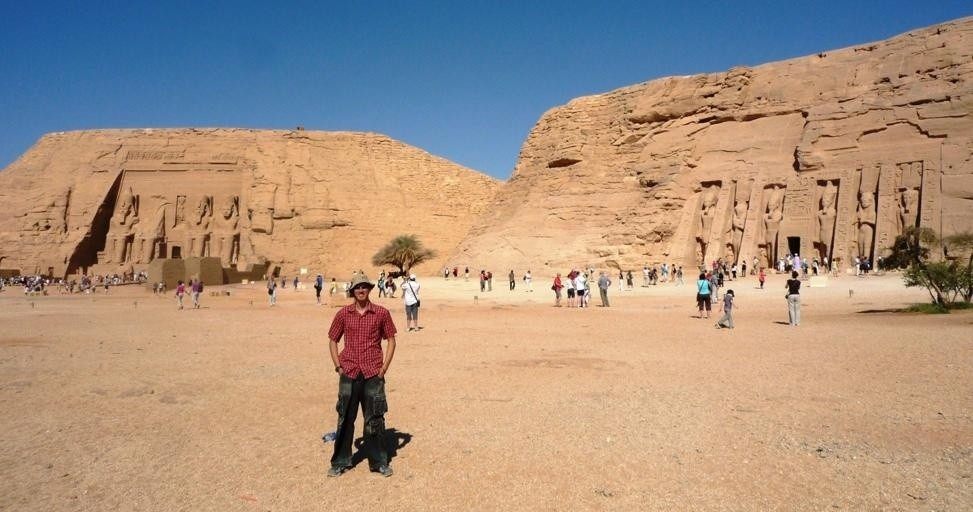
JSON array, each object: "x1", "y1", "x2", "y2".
[
  {"x1": 345, "y1": 272, "x2": 376, "y2": 293},
  {"x1": 409, "y1": 273, "x2": 416, "y2": 280}
]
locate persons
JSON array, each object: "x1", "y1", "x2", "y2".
[
  {"x1": 0, "y1": 272, "x2": 204, "y2": 310},
  {"x1": 696, "y1": 257, "x2": 739, "y2": 318},
  {"x1": 465, "y1": 266, "x2": 469, "y2": 280},
  {"x1": 445, "y1": 268, "x2": 449, "y2": 280},
  {"x1": 266, "y1": 270, "x2": 421, "y2": 331},
  {"x1": 329, "y1": 276, "x2": 397, "y2": 476},
  {"x1": 525, "y1": 270, "x2": 533, "y2": 292},
  {"x1": 487, "y1": 271, "x2": 492, "y2": 290},
  {"x1": 785, "y1": 271, "x2": 801, "y2": 326},
  {"x1": 619, "y1": 263, "x2": 684, "y2": 291},
  {"x1": 509, "y1": 270, "x2": 515, "y2": 290},
  {"x1": 480, "y1": 270, "x2": 487, "y2": 291},
  {"x1": 753, "y1": 256, "x2": 767, "y2": 288},
  {"x1": 855, "y1": 256, "x2": 883, "y2": 276},
  {"x1": 553, "y1": 268, "x2": 612, "y2": 308},
  {"x1": 776, "y1": 254, "x2": 839, "y2": 277},
  {"x1": 742, "y1": 260, "x2": 746, "y2": 277},
  {"x1": 453, "y1": 267, "x2": 458, "y2": 277},
  {"x1": 716, "y1": 289, "x2": 738, "y2": 329}
]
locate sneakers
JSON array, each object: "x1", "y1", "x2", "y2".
[
  {"x1": 374, "y1": 462, "x2": 395, "y2": 478},
  {"x1": 404, "y1": 327, "x2": 420, "y2": 332},
  {"x1": 326, "y1": 462, "x2": 350, "y2": 477}
]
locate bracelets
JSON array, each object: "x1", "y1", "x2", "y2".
[{"x1": 380, "y1": 368, "x2": 386, "y2": 374}]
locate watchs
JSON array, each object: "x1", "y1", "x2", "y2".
[{"x1": 335, "y1": 366, "x2": 342, "y2": 372}]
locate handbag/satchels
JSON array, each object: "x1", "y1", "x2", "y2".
[{"x1": 416, "y1": 298, "x2": 421, "y2": 307}]
